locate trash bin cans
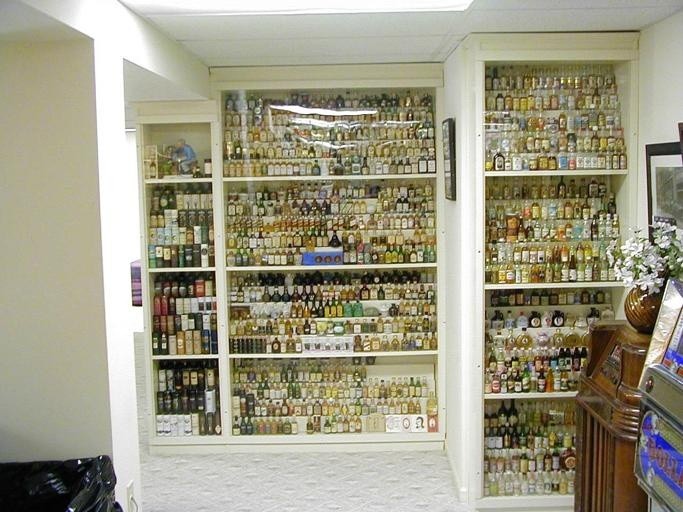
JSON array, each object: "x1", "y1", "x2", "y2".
[{"x1": 0, "y1": 454, "x2": 123, "y2": 512}]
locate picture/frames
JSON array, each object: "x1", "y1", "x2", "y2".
[{"x1": 644, "y1": 123, "x2": 683, "y2": 296}]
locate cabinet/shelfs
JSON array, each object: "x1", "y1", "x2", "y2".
[
  {"x1": 133, "y1": 98, "x2": 225, "y2": 446},
  {"x1": 440, "y1": 28, "x2": 641, "y2": 506},
  {"x1": 208, "y1": 58, "x2": 444, "y2": 448}
]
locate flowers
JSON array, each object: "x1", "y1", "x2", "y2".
[{"x1": 599, "y1": 220, "x2": 683, "y2": 295}]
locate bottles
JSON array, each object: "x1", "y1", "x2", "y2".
[
  {"x1": 485, "y1": 63, "x2": 628, "y2": 498},
  {"x1": 223, "y1": 89, "x2": 439, "y2": 434}
]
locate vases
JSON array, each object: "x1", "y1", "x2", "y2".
[{"x1": 624, "y1": 286, "x2": 662, "y2": 335}]
